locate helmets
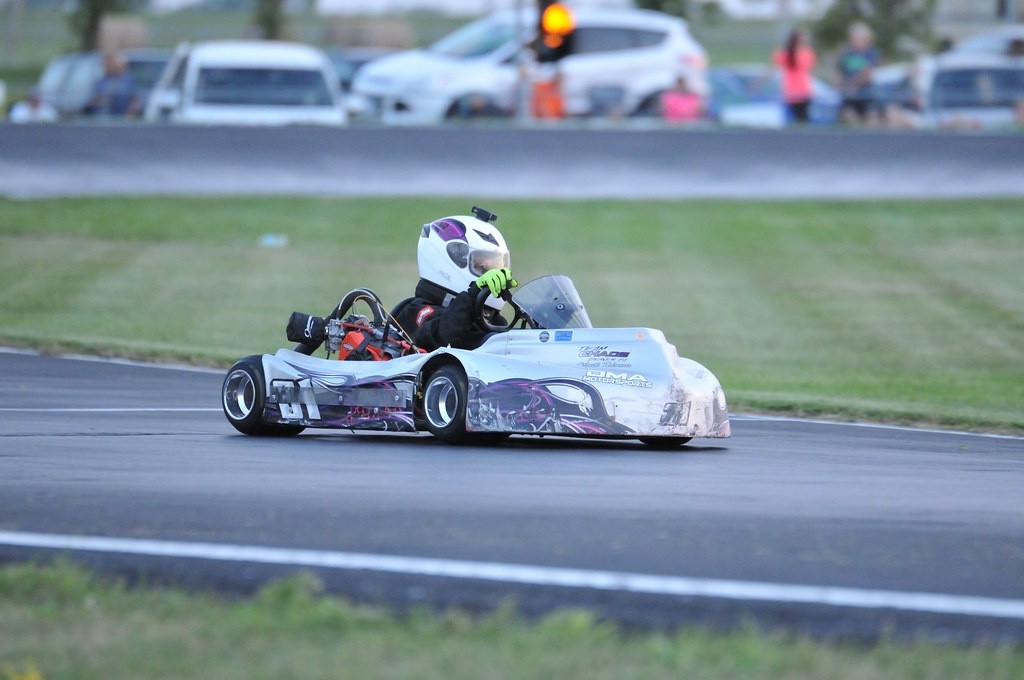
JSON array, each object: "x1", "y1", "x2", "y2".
[{"x1": 416, "y1": 215, "x2": 510, "y2": 311}]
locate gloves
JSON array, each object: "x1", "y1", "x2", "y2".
[{"x1": 469, "y1": 267, "x2": 518, "y2": 299}]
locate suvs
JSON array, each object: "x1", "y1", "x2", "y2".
[{"x1": 349, "y1": 0, "x2": 713, "y2": 123}]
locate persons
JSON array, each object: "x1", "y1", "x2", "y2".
[
  {"x1": 389, "y1": 207, "x2": 577, "y2": 353},
  {"x1": 90, "y1": 53, "x2": 142, "y2": 120},
  {"x1": 517, "y1": 28, "x2": 569, "y2": 119},
  {"x1": 658, "y1": 78, "x2": 704, "y2": 125},
  {"x1": 835, "y1": 24, "x2": 928, "y2": 129},
  {"x1": 775, "y1": 30, "x2": 818, "y2": 123},
  {"x1": 11, "y1": 89, "x2": 56, "y2": 121}
]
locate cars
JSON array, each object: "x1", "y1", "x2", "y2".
[
  {"x1": 9, "y1": 43, "x2": 403, "y2": 125},
  {"x1": 707, "y1": 21, "x2": 1024, "y2": 129}
]
